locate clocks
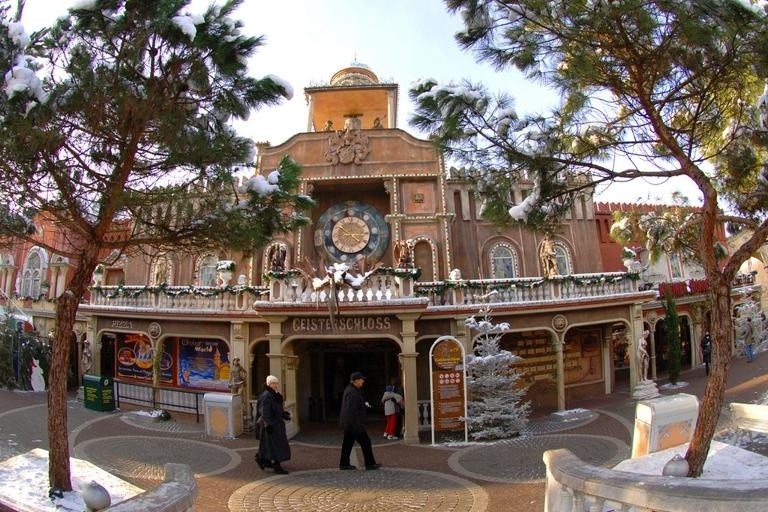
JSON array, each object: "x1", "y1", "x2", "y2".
[{"x1": 312, "y1": 200, "x2": 391, "y2": 268}]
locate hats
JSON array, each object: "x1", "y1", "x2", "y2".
[
  {"x1": 350, "y1": 372, "x2": 367, "y2": 381},
  {"x1": 266, "y1": 375, "x2": 279, "y2": 386}
]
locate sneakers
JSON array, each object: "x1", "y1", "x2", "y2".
[
  {"x1": 387, "y1": 435, "x2": 398, "y2": 440},
  {"x1": 384, "y1": 432, "x2": 388, "y2": 436}
]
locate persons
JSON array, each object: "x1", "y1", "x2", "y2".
[
  {"x1": 381, "y1": 384, "x2": 403, "y2": 441},
  {"x1": 226, "y1": 356, "x2": 248, "y2": 411},
  {"x1": 740, "y1": 317, "x2": 754, "y2": 363},
  {"x1": 637, "y1": 330, "x2": 651, "y2": 386},
  {"x1": 701, "y1": 331, "x2": 711, "y2": 376},
  {"x1": 337, "y1": 372, "x2": 382, "y2": 470},
  {"x1": 254, "y1": 374, "x2": 291, "y2": 474},
  {"x1": 390, "y1": 377, "x2": 404, "y2": 439},
  {"x1": 539, "y1": 231, "x2": 560, "y2": 276}
]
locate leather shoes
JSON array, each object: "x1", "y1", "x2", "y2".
[
  {"x1": 339, "y1": 464, "x2": 356, "y2": 470},
  {"x1": 365, "y1": 462, "x2": 382, "y2": 470},
  {"x1": 255, "y1": 452, "x2": 289, "y2": 474}
]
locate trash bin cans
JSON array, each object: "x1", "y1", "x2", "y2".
[
  {"x1": 203, "y1": 392, "x2": 244, "y2": 438},
  {"x1": 82, "y1": 373, "x2": 116, "y2": 412},
  {"x1": 631, "y1": 392, "x2": 701, "y2": 458}
]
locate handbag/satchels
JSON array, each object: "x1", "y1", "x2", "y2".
[{"x1": 702, "y1": 345, "x2": 711, "y2": 355}]
